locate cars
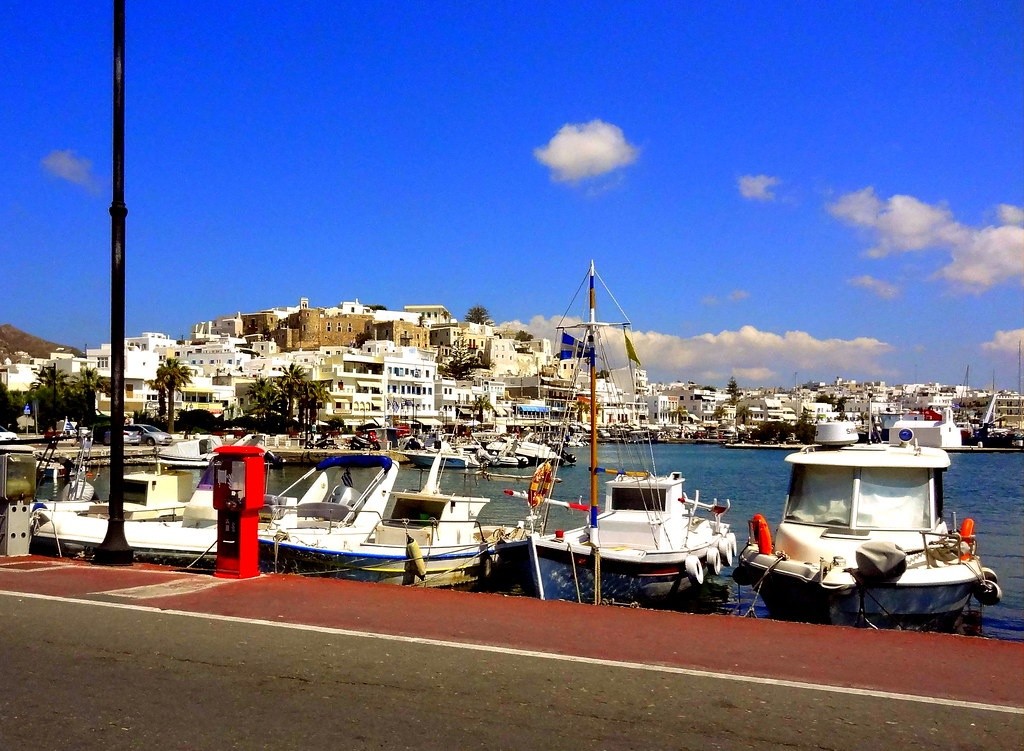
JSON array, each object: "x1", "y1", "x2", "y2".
[
  {"x1": 0, "y1": 425, "x2": 21, "y2": 442},
  {"x1": 130, "y1": 423, "x2": 173, "y2": 446},
  {"x1": 93, "y1": 421, "x2": 142, "y2": 447}
]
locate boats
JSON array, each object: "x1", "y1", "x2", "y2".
[
  {"x1": 744, "y1": 417, "x2": 1003, "y2": 637},
  {"x1": 27, "y1": 441, "x2": 365, "y2": 568},
  {"x1": 255, "y1": 451, "x2": 541, "y2": 590},
  {"x1": 156, "y1": 422, "x2": 751, "y2": 471}
]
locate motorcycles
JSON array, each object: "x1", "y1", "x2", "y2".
[
  {"x1": 306, "y1": 429, "x2": 330, "y2": 449},
  {"x1": 349, "y1": 426, "x2": 380, "y2": 451}
]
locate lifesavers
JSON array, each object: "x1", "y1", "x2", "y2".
[
  {"x1": 959, "y1": 518, "x2": 975, "y2": 560},
  {"x1": 527, "y1": 460, "x2": 553, "y2": 509},
  {"x1": 752, "y1": 513, "x2": 773, "y2": 553}
]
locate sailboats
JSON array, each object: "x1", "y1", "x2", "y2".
[{"x1": 504, "y1": 253, "x2": 739, "y2": 615}]
